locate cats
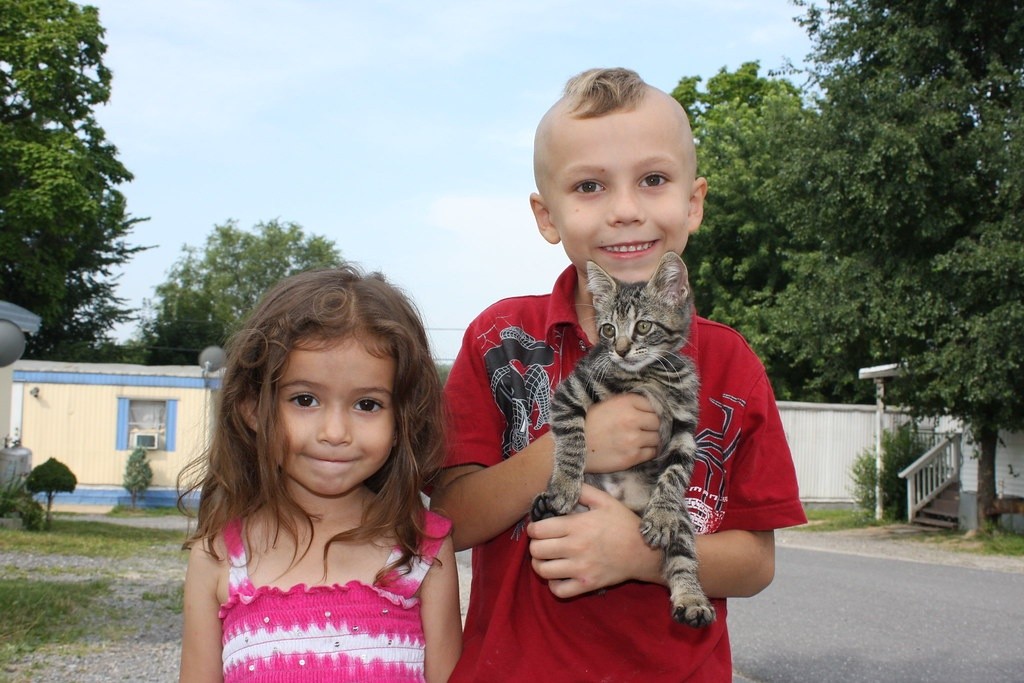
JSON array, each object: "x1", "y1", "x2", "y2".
[{"x1": 526, "y1": 250, "x2": 718, "y2": 632}]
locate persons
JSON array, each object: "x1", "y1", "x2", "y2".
[
  {"x1": 420, "y1": 68, "x2": 807, "y2": 683},
  {"x1": 177, "y1": 263, "x2": 463, "y2": 682}
]
